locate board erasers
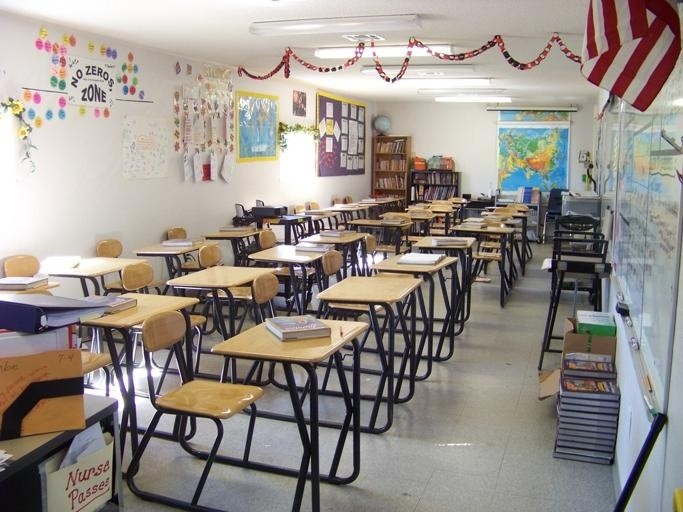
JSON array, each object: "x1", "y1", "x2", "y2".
[{"x1": 616, "y1": 303, "x2": 629, "y2": 316}]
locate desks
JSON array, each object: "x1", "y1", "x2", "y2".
[{"x1": 558, "y1": 192, "x2": 600, "y2": 217}]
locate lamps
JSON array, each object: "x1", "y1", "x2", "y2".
[
  {"x1": 247, "y1": 15, "x2": 420, "y2": 36},
  {"x1": 314, "y1": 44, "x2": 513, "y2": 103}
]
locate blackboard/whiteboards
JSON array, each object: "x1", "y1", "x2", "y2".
[{"x1": 594, "y1": 81, "x2": 682, "y2": 419}]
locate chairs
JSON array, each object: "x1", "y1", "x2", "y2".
[
  {"x1": 230, "y1": 197, "x2": 533, "y2": 308},
  {"x1": 2, "y1": 226, "x2": 480, "y2": 512},
  {"x1": 542, "y1": 187, "x2": 568, "y2": 244},
  {"x1": 537, "y1": 214, "x2": 611, "y2": 372}
]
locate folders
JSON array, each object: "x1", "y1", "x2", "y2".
[{"x1": 10, "y1": 293, "x2": 108, "y2": 333}]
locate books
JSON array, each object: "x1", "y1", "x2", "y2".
[
  {"x1": 295, "y1": 241, "x2": 336, "y2": 252},
  {"x1": 265, "y1": 314, "x2": 331, "y2": 342},
  {"x1": 396, "y1": 252, "x2": 447, "y2": 266},
  {"x1": 551, "y1": 358, "x2": 620, "y2": 466},
  {"x1": 432, "y1": 238, "x2": 468, "y2": 246},
  {"x1": 462, "y1": 222, "x2": 488, "y2": 229},
  {"x1": 304, "y1": 140, "x2": 458, "y2": 225},
  {"x1": 0, "y1": 276, "x2": 48, "y2": 290},
  {"x1": 219, "y1": 225, "x2": 256, "y2": 232},
  {"x1": 319, "y1": 228, "x2": 356, "y2": 238},
  {"x1": 484, "y1": 216, "x2": 508, "y2": 221},
  {"x1": 82, "y1": 294, "x2": 137, "y2": 315},
  {"x1": 516, "y1": 187, "x2": 538, "y2": 204},
  {"x1": 162, "y1": 238, "x2": 206, "y2": 247}
]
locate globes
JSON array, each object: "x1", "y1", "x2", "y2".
[{"x1": 374, "y1": 116, "x2": 391, "y2": 136}]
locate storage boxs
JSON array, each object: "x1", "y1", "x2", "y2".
[{"x1": 538, "y1": 316, "x2": 617, "y2": 402}]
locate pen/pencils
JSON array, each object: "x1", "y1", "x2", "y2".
[
  {"x1": 340, "y1": 326, "x2": 343, "y2": 336},
  {"x1": 643, "y1": 375, "x2": 652, "y2": 392}
]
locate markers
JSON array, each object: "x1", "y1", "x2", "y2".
[
  {"x1": 625, "y1": 316, "x2": 632, "y2": 327},
  {"x1": 631, "y1": 337, "x2": 638, "y2": 351},
  {"x1": 616, "y1": 292, "x2": 623, "y2": 302}
]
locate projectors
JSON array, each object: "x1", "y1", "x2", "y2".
[{"x1": 251, "y1": 205, "x2": 288, "y2": 217}]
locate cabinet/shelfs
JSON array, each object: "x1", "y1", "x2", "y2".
[
  {"x1": 408, "y1": 169, "x2": 462, "y2": 208},
  {"x1": 371, "y1": 135, "x2": 412, "y2": 211},
  {"x1": 495, "y1": 196, "x2": 540, "y2": 242}
]
